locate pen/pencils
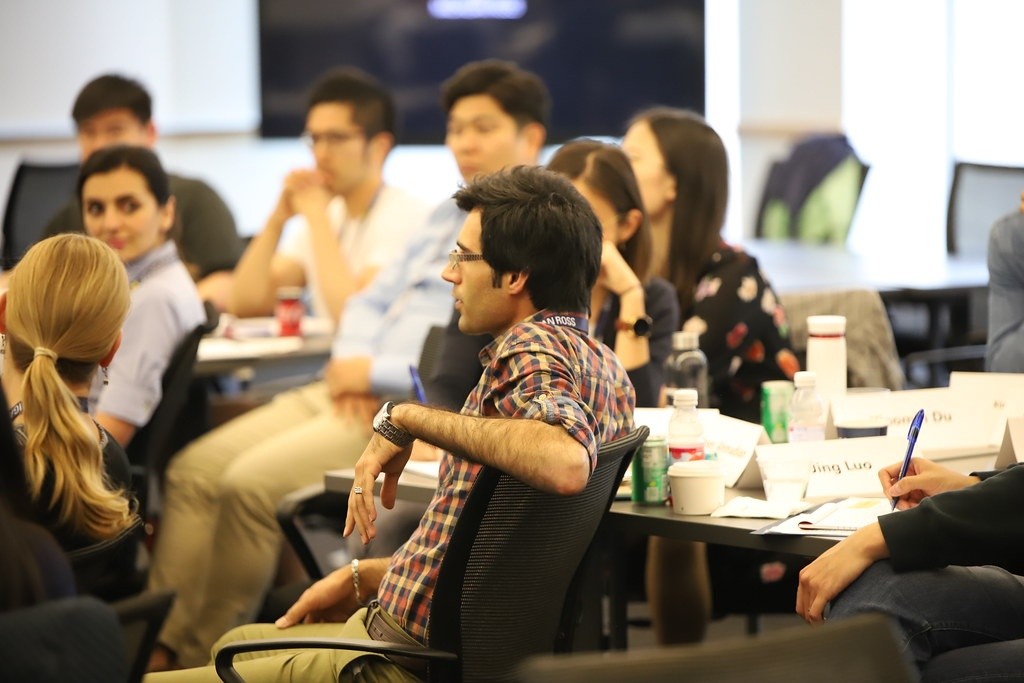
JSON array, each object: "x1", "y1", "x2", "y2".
[
  {"x1": 890, "y1": 408, "x2": 925, "y2": 510},
  {"x1": 409, "y1": 364, "x2": 428, "y2": 403}
]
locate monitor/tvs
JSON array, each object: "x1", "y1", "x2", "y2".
[{"x1": 258, "y1": 0, "x2": 705, "y2": 148}]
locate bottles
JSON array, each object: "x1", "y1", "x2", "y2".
[
  {"x1": 805, "y1": 314, "x2": 848, "y2": 399},
  {"x1": 784, "y1": 372, "x2": 826, "y2": 443},
  {"x1": 663, "y1": 332, "x2": 709, "y2": 408},
  {"x1": 665, "y1": 388, "x2": 705, "y2": 465},
  {"x1": 276, "y1": 283, "x2": 301, "y2": 336}
]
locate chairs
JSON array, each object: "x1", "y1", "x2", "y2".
[{"x1": 0, "y1": 155, "x2": 1024, "y2": 683}]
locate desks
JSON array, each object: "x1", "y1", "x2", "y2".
[
  {"x1": 186, "y1": 334, "x2": 332, "y2": 586},
  {"x1": 324, "y1": 381, "x2": 1024, "y2": 651}
]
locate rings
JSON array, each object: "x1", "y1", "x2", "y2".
[{"x1": 355, "y1": 487, "x2": 364, "y2": 495}]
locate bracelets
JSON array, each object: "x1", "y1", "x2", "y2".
[{"x1": 350, "y1": 558, "x2": 364, "y2": 605}]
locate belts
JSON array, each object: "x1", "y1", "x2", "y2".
[{"x1": 365, "y1": 600, "x2": 432, "y2": 678}]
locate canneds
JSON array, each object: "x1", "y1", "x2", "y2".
[
  {"x1": 630, "y1": 436, "x2": 670, "y2": 506},
  {"x1": 276, "y1": 293, "x2": 304, "y2": 335},
  {"x1": 760, "y1": 380, "x2": 793, "y2": 444}
]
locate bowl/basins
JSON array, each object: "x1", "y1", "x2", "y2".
[{"x1": 667, "y1": 459, "x2": 725, "y2": 515}]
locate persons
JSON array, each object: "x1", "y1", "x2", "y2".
[
  {"x1": 792, "y1": 457, "x2": 1024, "y2": 683},
  {"x1": 984, "y1": 189, "x2": 1024, "y2": 374},
  {"x1": 1, "y1": 60, "x2": 800, "y2": 683}
]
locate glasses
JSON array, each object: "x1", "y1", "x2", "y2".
[
  {"x1": 301, "y1": 128, "x2": 366, "y2": 149},
  {"x1": 449, "y1": 249, "x2": 485, "y2": 271}
]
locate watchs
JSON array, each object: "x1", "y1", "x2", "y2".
[
  {"x1": 616, "y1": 313, "x2": 655, "y2": 339},
  {"x1": 371, "y1": 399, "x2": 415, "y2": 448}
]
